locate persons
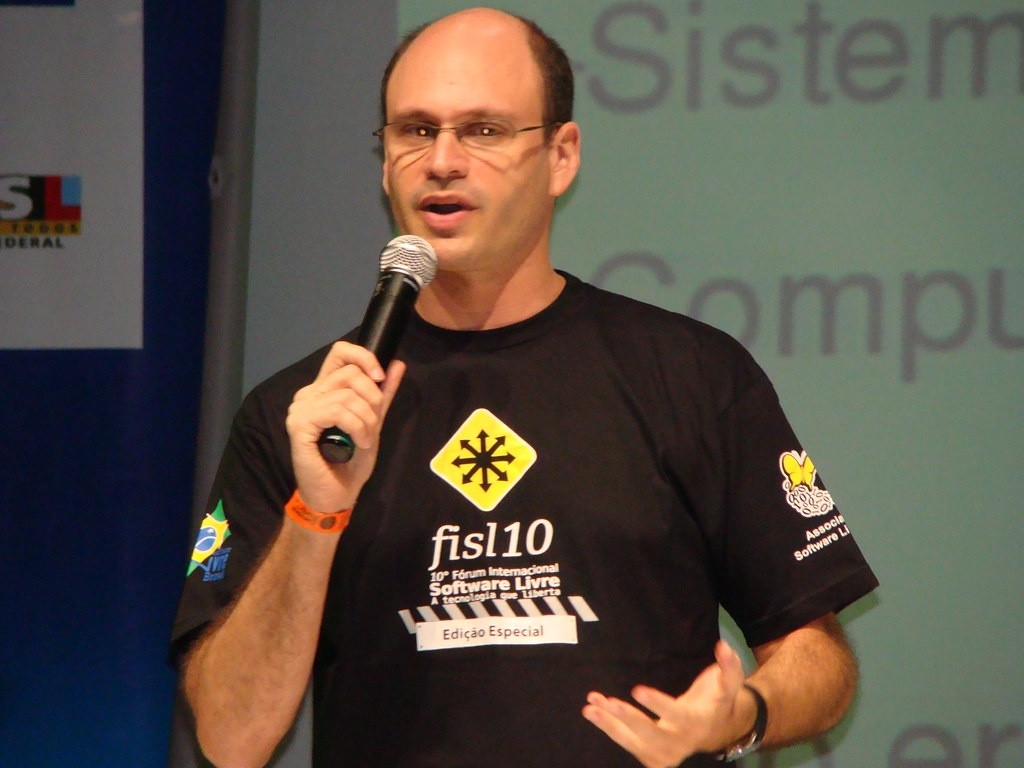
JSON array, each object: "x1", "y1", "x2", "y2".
[{"x1": 171, "y1": 6, "x2": 880, "y2": 768}]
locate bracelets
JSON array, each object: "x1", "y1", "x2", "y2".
[{"x1": 285, "y1": 491, "x2": 352, "y2": 533}]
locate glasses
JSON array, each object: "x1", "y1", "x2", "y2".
[{"x1": 373, "y1": 118, "x2": 563, "y2": 151}]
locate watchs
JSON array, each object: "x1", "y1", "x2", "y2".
[{"x1": 715, "y1": 686, "x2": 767, "y2": 762}]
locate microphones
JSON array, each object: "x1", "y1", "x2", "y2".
[{"x1": 318, "y1": 235, "x2": 438, "y2": 464}]
